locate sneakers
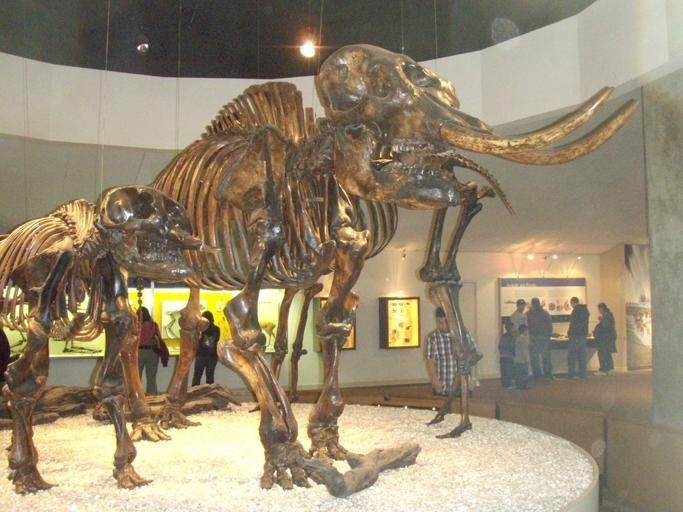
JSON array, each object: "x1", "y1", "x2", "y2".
[{"x1": 592, "y1": 368, "x2": 615, "y2": 376}]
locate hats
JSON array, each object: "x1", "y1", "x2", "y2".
[{"x1": 517, "y1": 299, "x2": 527, "y2": 305}]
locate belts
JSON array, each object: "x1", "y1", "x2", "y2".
[{"x1": 139, "y1": 344, "x2": 153, "y2": 350}]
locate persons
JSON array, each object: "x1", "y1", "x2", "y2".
[
  {"x1": 191, "y1": 310, "x2": 220, "y2": 386},
  {"x1": 523, "y1": 297, "x2": 554, "y2": 381},
  {"x1": 422, "y1": 306, "x2": 478, "y2": 399},
  {"x1": 497, "y1": 321, "x2": 516, "y2": 390},
  {"x1": 563, "y1": 296, "x2": 590, "y2": 381},
  {"x1": 592, "y1": 302, "x2": 618, "y2": 376},
  {"x1": 0, "y1": 318, "x2": 9, "y2": 395},
  {"x1": 507, "y1": 299, "x2": 528, "y2": 382},
  {"x1": 134, "y1": 306, "x2": 169, "y2": 397},
  {"x1": 512, "y1": 323, "x2": 532, "y2": 390}
]
